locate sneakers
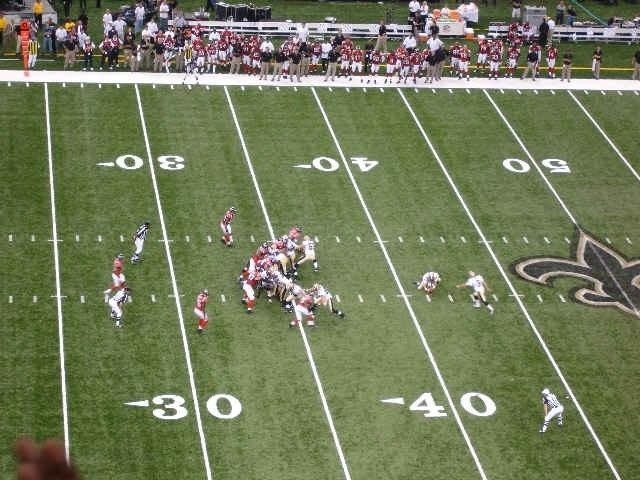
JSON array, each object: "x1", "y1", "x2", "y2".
[{"x1": 538, "y1": 421, "x2": 563, "y2": 434}]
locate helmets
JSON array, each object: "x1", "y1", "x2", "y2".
[
  {"x1": 203, "y1": 205, "x2": 320, "y2": 296},
  {"x1": 116, "y1": 205, "x2": 152, "y2": 294},
  {"x1": 429, "y1": 272, "x2": 550, "y2": 395}
]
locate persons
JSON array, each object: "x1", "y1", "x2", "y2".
[
  {"x1": 365, "y1": 21, "x2": 387, "y2": 84},
  {"x1": 184, "y1": 21, "x2": 362, "y2": 84},
  {"x1": 239, "y1": 224, "x2": 345, "y2": 329},
  {"x1": 99, "y1": 1, "x2": 181, "y2": 74},
  {"x1": 592, "y1": 46, "x2": 602, "y2": 78},
  {"x1": 1, "y1": 0, "x2": 96, "y2": 71},
  {"x1": 476, "y1": 0, "x2": 572, "y2": 84},
  {"x1": 194, "y1": 289, "x2": 209, "y2": 333},
  {"x1": 106, "y1": 253, "x2": 131, "y2": 328},
  {"x1": 221, "y1": 207, "x2": 239, "y2": 247},
  {"x1": 417, "y1": 271, "x2": 439, "y2": 294},
  {"x1": 632, "y1": 48, "x2": 640, "y2": 80},
  {"x1": 132, "y1": 221, "x2": 149, "y2": 263},
  {"x1": 538, "y1": 387, "x2": 565, "y2": 432},
  {"x1": 457, "y1": 271, "x2": 494, "y2": 315},
  {"x1": 555, "y1": 2, "x2": 577, "y2": 26},
  {"x1": 385, "y1": 1, "x2": 471, "y2": 85}
]
